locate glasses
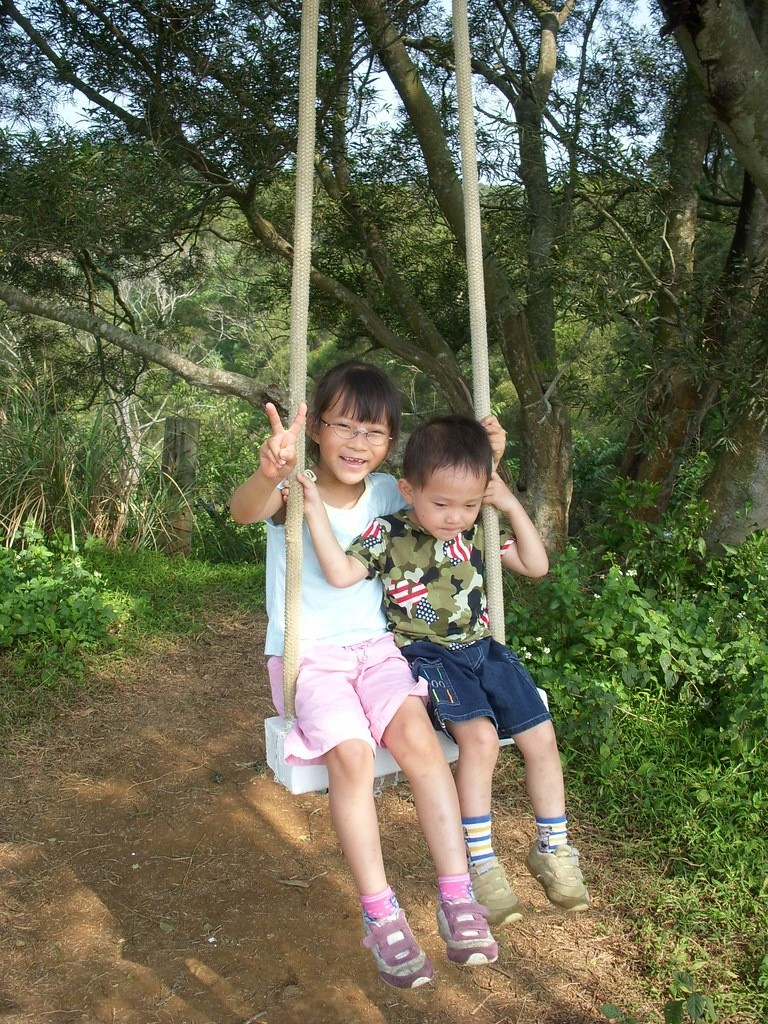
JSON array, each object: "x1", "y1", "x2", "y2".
[{"x1": 318, "y1": 415, "x2": 395, "y2": 446}]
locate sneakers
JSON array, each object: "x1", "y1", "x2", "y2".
[
  {"x1": 359, "y1": 904, "x2": 434, "y2": 990},
  {"x1": 468, "y1": 856, "x2": 526, "y2": 927},
  {"x1": 436, "y1": 896, "x2": 499, "y2": 967},
  {"x1": 523, "y1": 845, "x2": 590, "y2": 912}
]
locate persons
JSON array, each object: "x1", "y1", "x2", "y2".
[
  {"x1": 230, "y1": 361, "x2": 499, "y2": 987},
  {"x1": 283, "y1": 415, "x2": 590, "y2": 927}
]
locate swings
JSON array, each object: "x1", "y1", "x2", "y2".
[{"x1": 252, "y1": 0, "x2": 558, "y2": 799}]
